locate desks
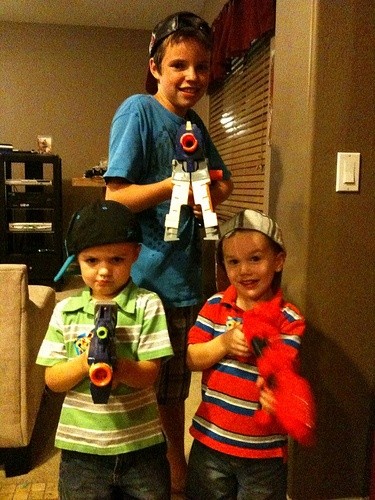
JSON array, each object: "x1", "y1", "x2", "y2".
[{"x1": 71, "y1": 178, "x2": 107, "y2": 199}]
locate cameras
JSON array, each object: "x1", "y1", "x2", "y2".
[{"x1": 85, "y1": 168, "x2": 106, "y2": 178}]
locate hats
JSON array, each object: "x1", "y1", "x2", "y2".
[
  {"x1": 54, "y1": 199, "x2": 141, "y2": 284},
  {"x1": 145, "y1": 11, "x2": 214, "y2": 95},
  {"x1": 215, "y1": 208, "x2": 288, "y2": 292}
]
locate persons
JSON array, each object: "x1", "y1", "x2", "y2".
[
  {"x1": 187, "y1": 207, "x2": 306, "y2": 500},
  {"x1": 35, "y1": 198, "x2": 177, "y2": 500},
  {"x1": 106, "y1": 11, "x2": 235, "y2": 500}
]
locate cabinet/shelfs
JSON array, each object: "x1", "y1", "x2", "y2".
[{"x1": 0, "y1": 155, "x2": 62, "y2": 286}]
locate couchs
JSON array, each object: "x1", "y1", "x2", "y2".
[{"x1": 0, "y1": 264, "x2": 55, "y2": 478}]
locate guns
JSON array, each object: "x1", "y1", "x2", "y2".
[
  {"x1": 164, "y1": 121, "x2": 223, "y2": 242},
  {"x1": 87, "y1": 300, "x2": 118, "y2": 404},
  {"x1": 239, "y1": 303, "x2": 317, "y2": 447}
]
